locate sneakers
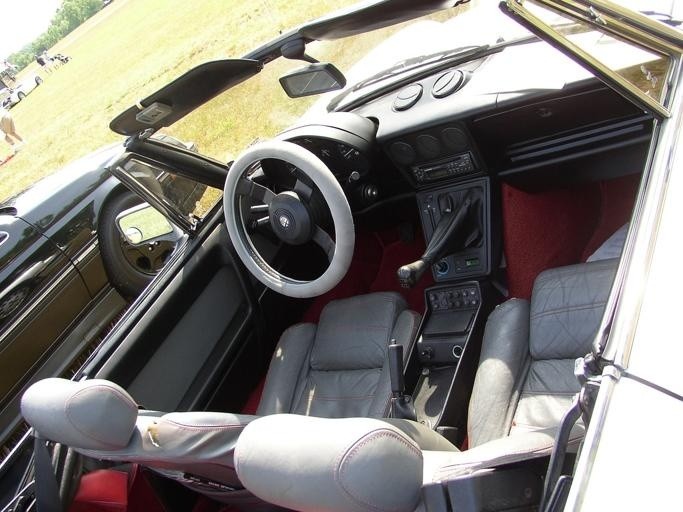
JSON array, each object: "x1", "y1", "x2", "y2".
[{"x1": 12, "y1": 140, "x2": 26, "y2": 152}]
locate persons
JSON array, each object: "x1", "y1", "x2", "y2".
[
  {"x1": 0, "y1": 106, "x2": 29, "y2": 153},
  {"x1": 3, "y1": 61, "x2": 15, "y2": 71},
  {"x1": 42, "y1": 51, "x2": 59, "y2": 71},
  {"x1": 33, "y1": 55, "x2": 53, "y2": 76}
]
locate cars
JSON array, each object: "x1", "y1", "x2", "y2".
[
  {"x1": 19, "y1": 1, "x2": 683, "y2": 509},
  {"x1": 3, "y1": 75, "x2": 42, "y2": 108},
  {"x1": 0, "y1": 134, "x2": 207, "y2": 512}
]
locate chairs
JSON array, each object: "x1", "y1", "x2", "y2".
[
  {"x1": 19, "y1": 270, "x2": 419, "y2": 512},
  {"x1": 234, "y1": 257, "x2": 621, "y2": 512}
]
